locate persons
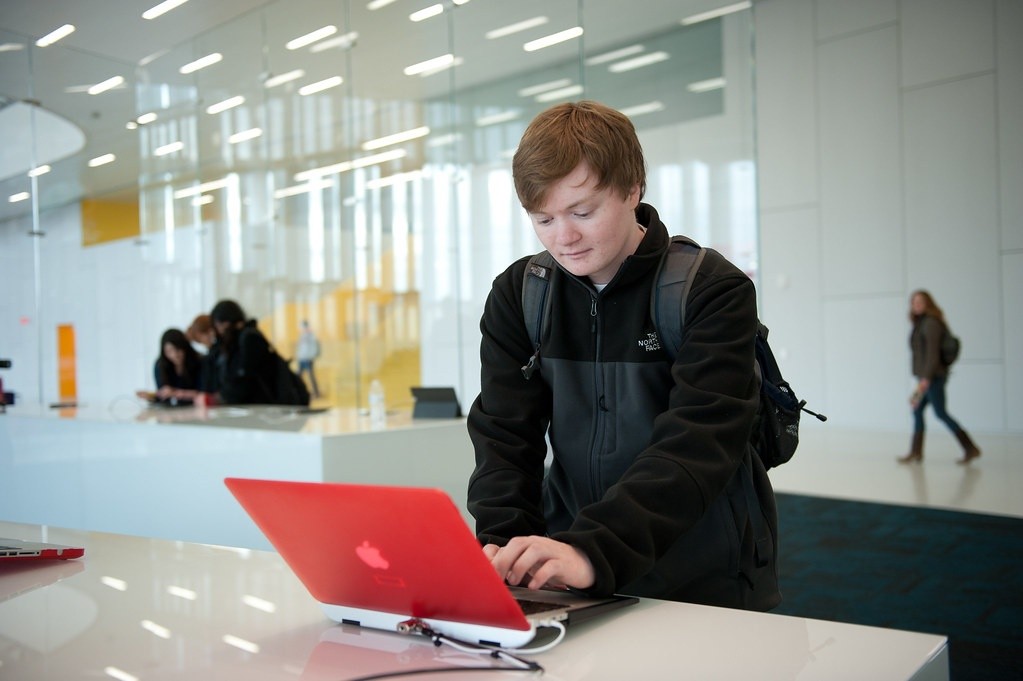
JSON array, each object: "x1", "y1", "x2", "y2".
[
  {"x1": 895, "y1": 290, "x2": 982, "y2": 466},
  {"x1": 153, "y1": 300, "x2": 325, "y2": 407},
  {"x1": 463, "y1": 106, "x2": 827, "y2": 612}
]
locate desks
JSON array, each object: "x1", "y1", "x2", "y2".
[
  {"x1": 0, "y1": 404, "x2": 477, "y2": 551},
  {"x1": 1, "y1": 521, "x2": 949, "y2": 681}
]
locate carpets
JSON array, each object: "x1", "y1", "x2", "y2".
[{"x1": 770, "y1": 492, "x2": 1023, "y2": 680}]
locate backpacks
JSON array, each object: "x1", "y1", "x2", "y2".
[
  {"x1": 522, "y1": 235, "x2": 826, "y2": 473},
  {"x1": 941, "y1": 331, "x2": 959, "y2": 366}
]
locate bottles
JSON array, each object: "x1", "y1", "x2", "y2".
[{"x1": 368, "y1": 379, "x2": 387, "y2": 427}]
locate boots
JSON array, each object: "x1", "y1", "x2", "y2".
[
  {"x1": 956, "y1": 430, "x2": 980, "y2": 463},
  {"x1": 898, "y1": 431, "x2": 922, "y2": 462}
]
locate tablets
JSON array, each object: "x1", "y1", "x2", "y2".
[{"x1": 410, "y1": 387, "x2": 462, "y2": 417}]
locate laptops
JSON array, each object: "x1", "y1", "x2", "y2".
[
  {"x1": 0, "y1": 538, "x2": 85, "y2": 560},
  {"x1": 222, "y1": 477, "x2": 641, "y2": 646}
]
